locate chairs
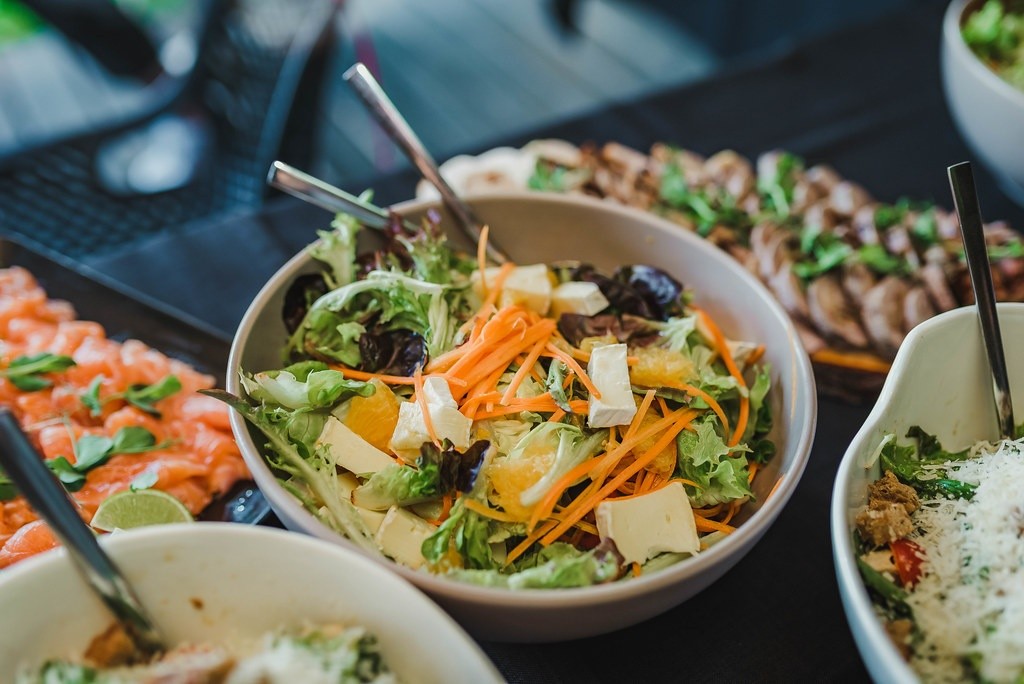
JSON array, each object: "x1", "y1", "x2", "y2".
[{"x1": 1, "y1": 0, "x2": 340, "y2": 259}]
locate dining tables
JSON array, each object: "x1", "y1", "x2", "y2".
[{"x1": 1, "y1": 0, "x2": 1022, "y2": 683}]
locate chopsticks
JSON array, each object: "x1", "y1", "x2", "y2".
[{"x1": 268, "y1": 62, "x2": 508, "y2": 267}]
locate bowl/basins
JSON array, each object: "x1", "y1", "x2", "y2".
[
  {"x1": 224, "y1": 190, "x2": 816, "y2": 638},
  {"x1": 832, "y1": 300, "x2": 1024, "y2": 681},
  {"x1": 944, "y1": 0, "x2": 1023, "y2": 199},
  {"x1": 3, "y1": 520, "x2": 508, "y2": 684}
]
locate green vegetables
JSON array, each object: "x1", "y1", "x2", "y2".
[
  {"x1": 198, "y1": 158, "x2": 1022, "y2": 590},
  {"x1": 1, "y1": 356, "x2": 182, "y2": 496}
]
